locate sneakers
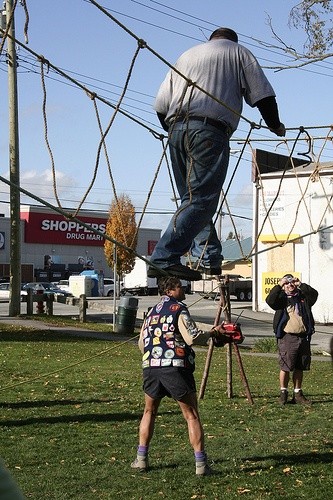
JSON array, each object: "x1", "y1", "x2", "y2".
[
  {"x1": 293, "y1": 390, "x2": 313, "y2": 405},
  {"x1": 196, "y1": 463, "x2": 218, "y2": 475},
  {"x1": 131, "y1": 455, "x2": 148, "y2": 470},
  {"x1": 147, "y1": 263, "x2": 202, "y2": 281},
  {"x1": 280, "y1": 390, "x2": 288, "y2": 404},
  {"x1": 202, "y1": 268, "x2": 221, "y2": 275}
]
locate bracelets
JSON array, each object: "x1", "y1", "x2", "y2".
[{"x1": 213, "y1": 329, "x2": 218, "y2": 339}]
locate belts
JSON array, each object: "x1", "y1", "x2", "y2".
[{"x1": 169, "y1": 116, "x2": 229, "y2": 134}]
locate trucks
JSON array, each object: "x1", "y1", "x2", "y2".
[{"x1": 122, "y1": 255, "x2": 189, "y2": 296}]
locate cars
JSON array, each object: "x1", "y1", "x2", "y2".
[
  {"x1": 0, "y1": 283, "x2": 28, "y2": 303},
  {"x1": 51, "y1": 269, "x2": 125, "y2": 297},
  {"x1": 23, "y1": 282, "x2": 73, "y2": 302}
]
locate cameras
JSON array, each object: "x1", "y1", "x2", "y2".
[{"x1": 287, "y1": 278, "x2": 293, "y2": 282}]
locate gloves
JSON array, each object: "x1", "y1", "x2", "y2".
[{"x1": 270, "y1": 123, "x2": 285, "y2": 136}]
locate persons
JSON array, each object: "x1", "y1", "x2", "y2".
[
  {"x1": 265, "y1": 274, "x2": 319, "y2": 404},
  {"x1": 130, "y1": 277, "x2": 237, "y2": 476},
  {"x1": 146, "y1": 28, "x2": 286, "y2": 282}
]
